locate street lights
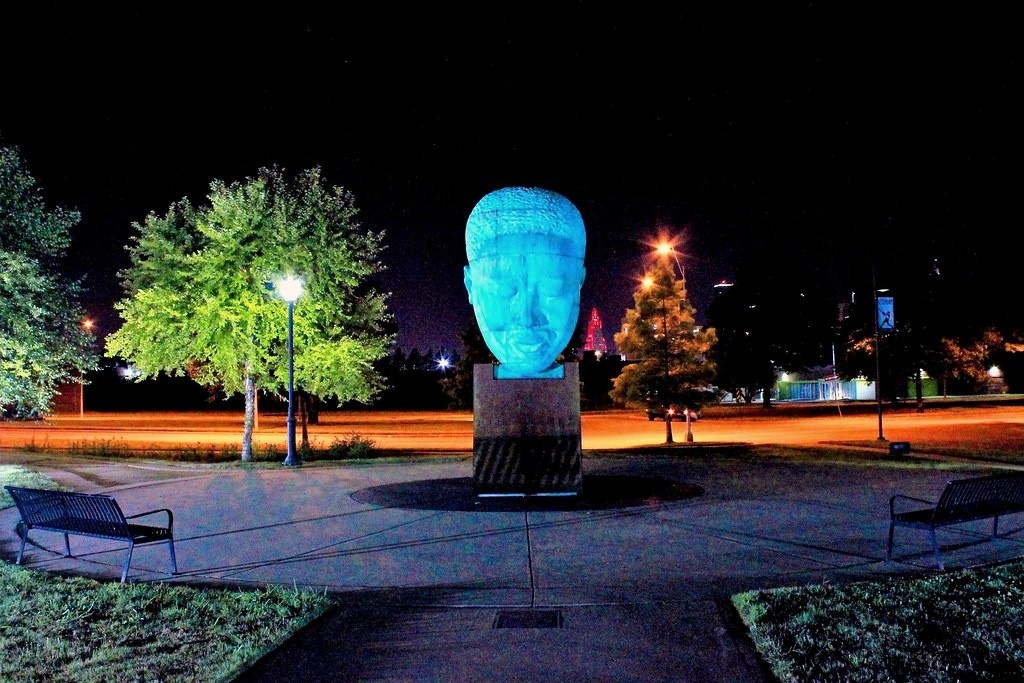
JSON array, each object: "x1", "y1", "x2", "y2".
[
  {"x1": 874, "y1": 284, "x2": 892, "y2": 444},
  {"x1": 640, "y1": 233, "x2": 679, "y2": 443},
  {"x1": 276, "y1": 277, "x2": 308, "y2": 468}
]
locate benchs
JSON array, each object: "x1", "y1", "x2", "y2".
[
  {"x1": 887, "y1": 470, "x2": 1024, "y2": 574},
  {"x1": 3, "y1": 486, "x2": 177, "y2": 585}
]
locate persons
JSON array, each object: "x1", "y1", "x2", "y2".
[{"x1": 463, "y1": 186, "x2": 587, "y2": 380}]
